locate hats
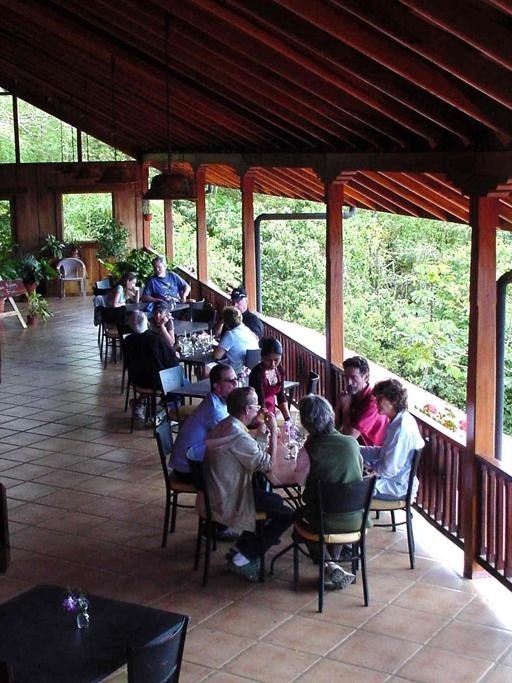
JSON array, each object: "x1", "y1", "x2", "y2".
[{"x1": 229, "y1": 288, "x2": 247, "y2": 298}]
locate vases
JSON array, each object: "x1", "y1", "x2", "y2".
[
  {"x1": 284, "y1": 444, "x2": 299, "y2": 459},
  {"x1": 76, "y1": 612, "x2": 90, "y2": 629},
  {"x1": 237, "y1": 378, "x2": 248, "y2": 386}
]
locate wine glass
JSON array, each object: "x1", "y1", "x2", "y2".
[
  {"x1": 179, "y1": 337, "x2": 187, "y2": 352},
  {"x1": 285, "y1": 439, "x2": 296, "y2": 460}
]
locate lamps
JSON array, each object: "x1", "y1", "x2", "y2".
[
  {"x1": 97, "y1": 26, "x2": 137, "y2": 185},
  {"x1": 142, "y1": 25, "x2": 199, "y2": 201},
  {"x1": 68, "y1": 33, "x2": 103, "y2": 188}
]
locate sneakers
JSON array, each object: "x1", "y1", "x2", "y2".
[
  {"x1": 327, "y1": 564, "x2": 356, "y2": 589},
  {"x1": 226, "y1": 553, "x2": 270, "y2": 583},
  {"x1": 202, "y1": 525, "x2": 240, "y2": 542},
  {"x1": 150, "y1": 409, "x2": 164, "y2": 426},
  {"x1": 129, "y1": 398, "x2": 145, "y2": 423}
]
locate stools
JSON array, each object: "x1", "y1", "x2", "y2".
[{"x1": 128, "y1": 385, "x2": 162, "y2": 423}]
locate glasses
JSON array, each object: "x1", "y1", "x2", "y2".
[
  {"x1": 222, "y1": 377, "x2": 240, "y2": 383},
  {"x1": 250, "y1": 404, "x2": 261, "y2": 412}
]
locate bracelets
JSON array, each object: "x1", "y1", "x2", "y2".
[{"x1": 159, "y1": 327, "x2": 167, "y2": 337}]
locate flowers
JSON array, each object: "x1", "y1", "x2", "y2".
[
  {"x1": 62, "y1": 586, "x2": 88, "y2": 612},
  {"x1": 284, "y1": 419, "x2": 308, "y2": 447},
  {"x1": 236, "y1": 365, "x2": 251, "y2": 379}
]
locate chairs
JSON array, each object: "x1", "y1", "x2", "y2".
[
  {"x1": 190, "y1": 297, "x2": 217, "y2": 327},
  {"x1": 364, "y1": 437, "x2": 430, "y2": 569},
  {"x1": 116, "y1": 325, "x2": 134, "y2": 394},
  {"x1": 155, "y1": 420, "x2": 218, "y2": 552},
  {"x1": 246, "y1": 348, "x2": 262, "y2": 370},
  {"x1": 187, "y1": 458, "x2": 281, "y2": 587},
  {"x1": 292, "y1": 476, "x2": 380, "y2": 613},
  {"x1": 95, "y1": 279, "x2": 114, "y2": 293},
  {"x1": 159, "y1": 365, "x2": 184, "y2": 434},
  {"x1": 306, "y1": 370, "x2": 319, "y2": 396},
  {"x1": 95, "y1": 294, "x2": 120, "y2": 369},
  {"x1": 56, "y1": 258, "x2": 85, "y2": 298}
]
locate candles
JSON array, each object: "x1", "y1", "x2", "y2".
[
  {"x1": 209, "y1": 329, "x2": 213, "y2": 342},
  {"x1": 184, "y1": 330, "x2": 188, "y2": 340}
]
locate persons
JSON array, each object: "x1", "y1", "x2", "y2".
[
  {"x1": 102, "y1": 269, "x2": 140, "y2": 325},
  {"x1": 120, "y1": 309, "x2": 180, "y2": 427},
  {"x1": 197, "y1": 288, "x2": 265, "y2": 382},
  {"x1": 290, "y1": 394, "x2": 372, "y2": 591},
  {"x1": 150, "y1": 300, "x2": 175, "y2": 350},
  {"x1": 167, "y1": 364, "x2": 241, "y2": 543},
  {"x1": 142, "y1": 256, "x2": 192, "y2": 320},
  {"x1": 248, "y1": 338, "x2": 290, "y2": 431},
  {"x1": 333, "y1": 356, "x2": 391, "y2": 447},
  {"x1": 213, "y1": 305, "x2": 260, "y2": 385},
  {"x1": 194, "y1": 387, "x2": 296, "y2": 585},
  {"x1": 338, "y1": 379, "x2": 425, "y2": 561}
]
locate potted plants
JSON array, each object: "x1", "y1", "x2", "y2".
[
  {"x1": 16, "y1": 252, "x2": 45, "y2": 296},
  {"x1": 27, "y1": 288, "x2": 54, "y2": 326}
]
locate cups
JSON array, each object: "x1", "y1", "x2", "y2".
[
  {"x1": 194, "y1": 340, "x2": 202, "y2": 350},
  {"x1": 202, "y1": 338, "x2": 209, "y2": 350},
  {"x1": 184, "y1": 341, "x2": 194, "y2": 357}
]
